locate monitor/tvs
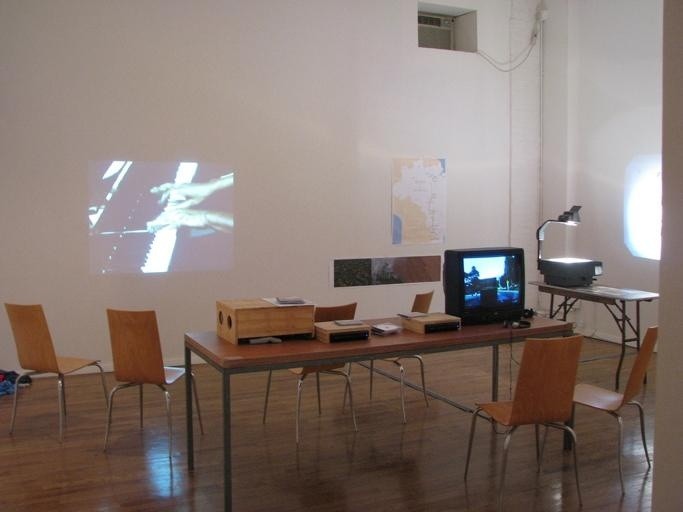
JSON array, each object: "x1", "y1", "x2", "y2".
[{"x1": 443, "y1": 247, "x2": 524, "y2": 325}]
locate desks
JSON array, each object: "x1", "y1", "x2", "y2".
[
  {"x1": 529, "y1": 277, "x2": 659, "y2": 394},
  {"x1": 183, "y1": 310, "x2": 574, "y2": 511}
]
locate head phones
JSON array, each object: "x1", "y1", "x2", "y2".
[{"x1": 502, "y1": 320, "x2": 529, "y2": 329}]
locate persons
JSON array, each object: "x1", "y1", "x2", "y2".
[{"x1": 469, "y1": 266, "x2": 477, "y2": 286}]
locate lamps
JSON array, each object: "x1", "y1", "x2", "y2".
[{"x1": 535, "y1": 203, "x2": 602, "y2": 287}]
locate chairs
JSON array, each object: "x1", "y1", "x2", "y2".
[
  {"x1": 537, "y1": 326, "x2": 658, "y2": 494},
  {"x1": 463, "y1": 334, "x2": 584, "y2": 509},
  {"x1": 342, "y1": 289, "x2": 436, "y2": 423},
  {"x1": 4, "y1": 302, "x2": 111, "y2": 436},
  {"x1": 101, "y1": 305, "x2": 204, "y2": 440},
  {"x1": 260, "y1": 304, "x2": 361, "y2": 439}
]
